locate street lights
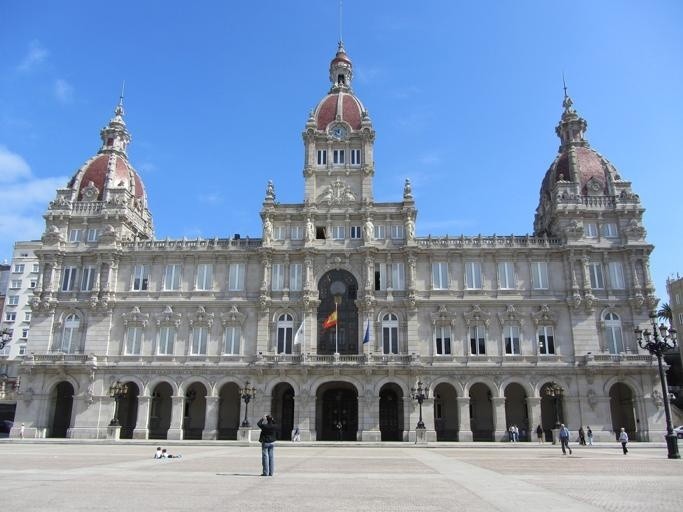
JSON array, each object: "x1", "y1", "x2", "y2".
[
  {"x1": 106, "y1": 380, "x2": 129, "y2": 426},
  {"x1": 543, "y1": 377, "x2": 565, "y2": 428},
  {"x1": 238, "y1": 380, "x2": 256, "y2": 429},
  {"x1": 409, "y1": 379, "x2": 430, "y2": 429},
  {"x1": 633, "y1": 306, "x2": 683, "y2": 461}
]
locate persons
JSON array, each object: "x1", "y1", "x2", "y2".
[
  {"x1": 294, "y1": 427, "x2": 300, "y2": 441},
  {"x1": 537, "y1": 425, "x2": 544, "y2": 444},
  {"x1": 586, "y1": 426, "x2": 593, "y2": 446},
  {"x1": 508, "y1": 423, "x2": 528, "y2": 442},
  {"x1": 154, "y1": 447, "x2": 181, "y2": 459},
  {"x1": 559, "y1": 423, "x2": 572, "y2": 455},
  {"x1": 257, "y1": 415, "x2": 277, "y2": 476},
  {"x1": 619, "y1": 427, "x2": 629, "y2": 454},
  {"x1": 335, "y1": 421, "x2": 343, "y2": 440},
  {"x1": 579, "y1": 427, "x2": 586, "y2": 445}
]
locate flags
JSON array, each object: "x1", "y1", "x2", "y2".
[
  {"x1": 323, "y1": 310, "x2": 337, "y2": 329},
  {"x1": 294, "y1": 321, "x2": 305, "y2": 344},
  {"x1": 362, "y1": 321, "x2": 369, "y2": 344}
]
